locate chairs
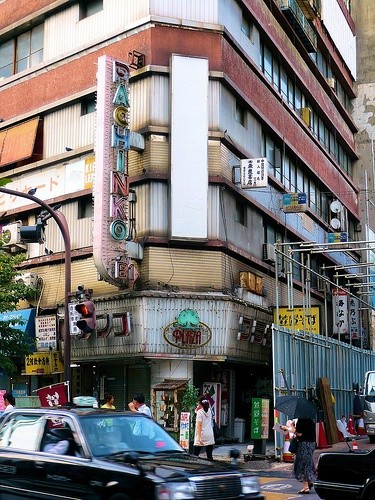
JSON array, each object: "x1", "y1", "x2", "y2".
[{"x1": 45, "y1": 428, "x2": 73, "y2": 443}]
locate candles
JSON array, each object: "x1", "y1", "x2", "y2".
[{"x1": 179, "y1": 383, "x2": 200, "y2": 455}]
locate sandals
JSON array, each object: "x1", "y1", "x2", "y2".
[
  {"x1": 297, "y1": 488, "x2": 310, "y2": 494},
  {"x1": 308, "y1": 483, "x2": 313, "y2": 490}
]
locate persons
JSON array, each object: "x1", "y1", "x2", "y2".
[
  {"x1": 280, "y1": 419, "x2": 297, "y2": 439},
  {"x1": 193, "y1": 397, "x2": 215, "y2": 460},
  {"x1": 128, "y1": 393, "x2": 152, "y2": 441},
  {"x1": 100, "y1": 395, "x2": 116, "y2": 430},
  {"x1": 295, "y1": 418, "x2": 316, "y2": 493},
  {"x1": 3, "y1": 393, "x2": 15, "y2": 412}
]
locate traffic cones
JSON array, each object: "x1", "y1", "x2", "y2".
[
  {"x1": 357, "y1": 412, "x2": 367, "y2": 436},
  {"x1": 349, "y1": 413, "x2": 356, "y2": 435},
  {"x1": 352, "y1": 441, "x2": 359, "y2": 450},
  {"x1": 317, "y1": 420, "x2": 332, "y2": 449},
  {"x1": 337, "y1": 428, "x2": 344, "y2": 441},
  {"x1": 281, "y1": 432, "x2": 294, "y2": 462}
]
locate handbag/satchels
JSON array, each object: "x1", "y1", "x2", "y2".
[
  {"x1": 210, "y1": 410, "x2": 221, "y2": 439},
  {"x1": 97, "y1": 418, "x2": 105, "y2": 428},
  {"x1": 289, "y1": 432, "x2": 302, "y2": 453}
]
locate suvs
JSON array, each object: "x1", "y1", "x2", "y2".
[{"x1": 0, "y1": 406, "x2": 267, "y2": 500}]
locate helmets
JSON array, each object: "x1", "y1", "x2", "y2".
[
  {"x1": 98, "y1": 419, "x2": 128, "y2": 448},
  {"x1": 46, "y1": 421, "x2": 73, "y2": 443}
]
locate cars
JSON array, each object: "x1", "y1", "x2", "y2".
[{"x1": 311, "y1": 438, "x2": 375, "y2": 500}]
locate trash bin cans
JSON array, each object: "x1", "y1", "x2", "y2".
[{"x1": 234, "y1": 419, "x2": 246, "y2": 443}]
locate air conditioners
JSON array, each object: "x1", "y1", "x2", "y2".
[
  {"x1": 2, "y1": 223, "x2": 23, "y2": 246},
  {"x1": 317, "y1": 275, "x2": 330, "y2": 293},
  {"x1": 262, "y1": 243, "x2": 275, "y2": 263},
  {"x1": 15, "y1": 272, "x2": 38, "y2": 288}
]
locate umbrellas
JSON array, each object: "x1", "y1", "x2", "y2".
[{"x1": 274, "y1": 395, "x2": 317, "y2": 420}]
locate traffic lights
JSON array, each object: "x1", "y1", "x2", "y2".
[{"x1": 75, "y1": 301, "x2": 98, "y2": 339}]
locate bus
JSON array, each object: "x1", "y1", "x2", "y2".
[{"x1": 363, "y1": 371, "x2": 375, "y2": 442}]
